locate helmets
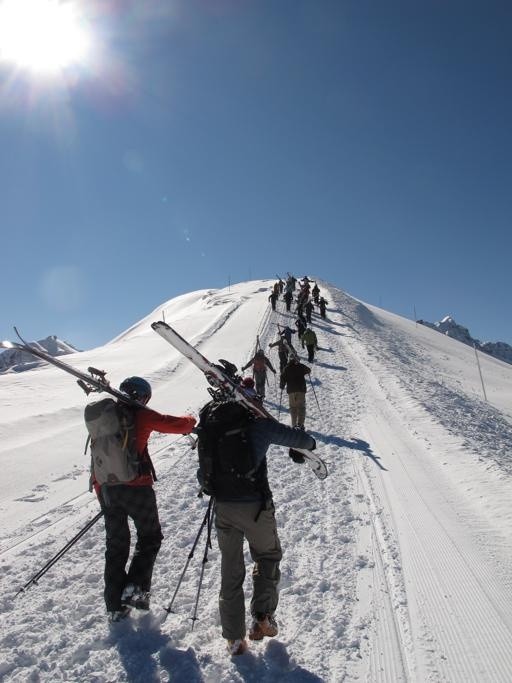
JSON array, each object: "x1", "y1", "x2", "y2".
[{"x1": 120, "y1": 377, "x2": 151, "y2": 402}]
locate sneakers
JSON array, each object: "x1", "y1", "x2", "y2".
[
  {"x1": 248, "y1": 612, "x2": 278, "y2": 640},
  {"x1": 108, "y1": 605, "x2": 132, "y2": 624},
  {"x1": 119, "y1": 583, "x2": 151, "y2": 610},
  {"x1": 226, "y1": 636, "x2": 247, "y2": 655}
]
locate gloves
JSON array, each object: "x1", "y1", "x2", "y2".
[{"x1": 290, "y1": 436, "x2": 316, "y2": 464}]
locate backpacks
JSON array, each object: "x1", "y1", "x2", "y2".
[
  {"x1": 84, "y1": 396, "x2": 153, "y2": 488},
  {"x1": 196, "y1": 399, "x2": 269, "y2": 499}
]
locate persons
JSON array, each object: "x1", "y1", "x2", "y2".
[
  {"x1": 241, "y1": 348, "x2": 277, "y2": 401},
  {"x1": 294, "y1": 313, "x2": 307, "y2": 339},
  {"x1": 93, "y1": 376, "x2": 198, "y2": 622},
  {"x1": 268, "y1": 335, "x2": 299, "y2": 374},
  {"x1": 268, "y1": 272, "x2": 328, "y2": 322},
  {"x1": 301, "y1": 326, "x2": 318, "y2": 363},
  {"x1": 198, "y1": 376, "x2": 317, "y2": 656},
  {"x1": 243, "y1": 377, "x2": 265, "y2": 405},
  {"x1": 279, "y1": 352, "x2": 312, "y2": 430},
  {"x1": 278, "y1": 324, "x2": 296, "y2": 344}
]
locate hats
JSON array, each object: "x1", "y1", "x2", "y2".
[{"x1": 237, "y1": 377, "x2": 264, "y2": 399}]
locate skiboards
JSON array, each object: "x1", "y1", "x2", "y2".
[
  {"x1": 282, "y1": 339, "x2": 301, "y2": 364},
  {"x1": 152, "y1": 321, "x2": 331, "y2": 480},
  {"x1": 1, "y1": 326, "x2": 203, "y2": 450},
  {"x1": 275, "y1": 271, "x2": 302, "y2": 288}
]
